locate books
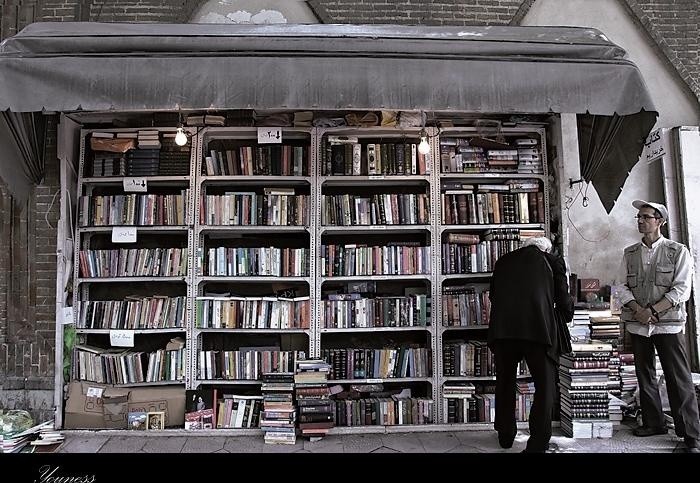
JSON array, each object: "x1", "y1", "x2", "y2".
[
  {"x1": 73, "y1": 111, "x2": 663, "y2": 446},
  {"x1": 1, "y1": 417, "x2": 66, "y2": 453}
]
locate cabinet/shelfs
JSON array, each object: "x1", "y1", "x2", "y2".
[{"x1": 52, "y1": 108, "x2": 568, "y2": 433}]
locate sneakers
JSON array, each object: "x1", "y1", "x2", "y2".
[
  {"x1": 633, "y1": 424, "x2": 668, "y2": 437},
  {"x1": 687, "y1": 445, "x2": 699, "y2": 452}
]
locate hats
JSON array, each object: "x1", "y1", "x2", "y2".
[{"x1": 632, "y1": 199, "x2": 669, "y2": 223}]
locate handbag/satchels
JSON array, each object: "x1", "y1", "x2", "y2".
[{"x1": 554, "y1": 307, "x2": 572, "y2": 354}]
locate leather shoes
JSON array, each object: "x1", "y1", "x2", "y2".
[{"x1": 543, "y1": 442, "x2": 559, "y2": 453}]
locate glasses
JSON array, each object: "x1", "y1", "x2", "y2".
[{"x1": 634, "y1": 215, "x2": 661, "y2": 222}]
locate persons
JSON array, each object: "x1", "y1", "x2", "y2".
[
  {"x1": 613, "y1": 197, "x2": 700, "y2": 456},
  {"x1": 484, "y1": 234, "x2": 576, "y2": 454}
]
